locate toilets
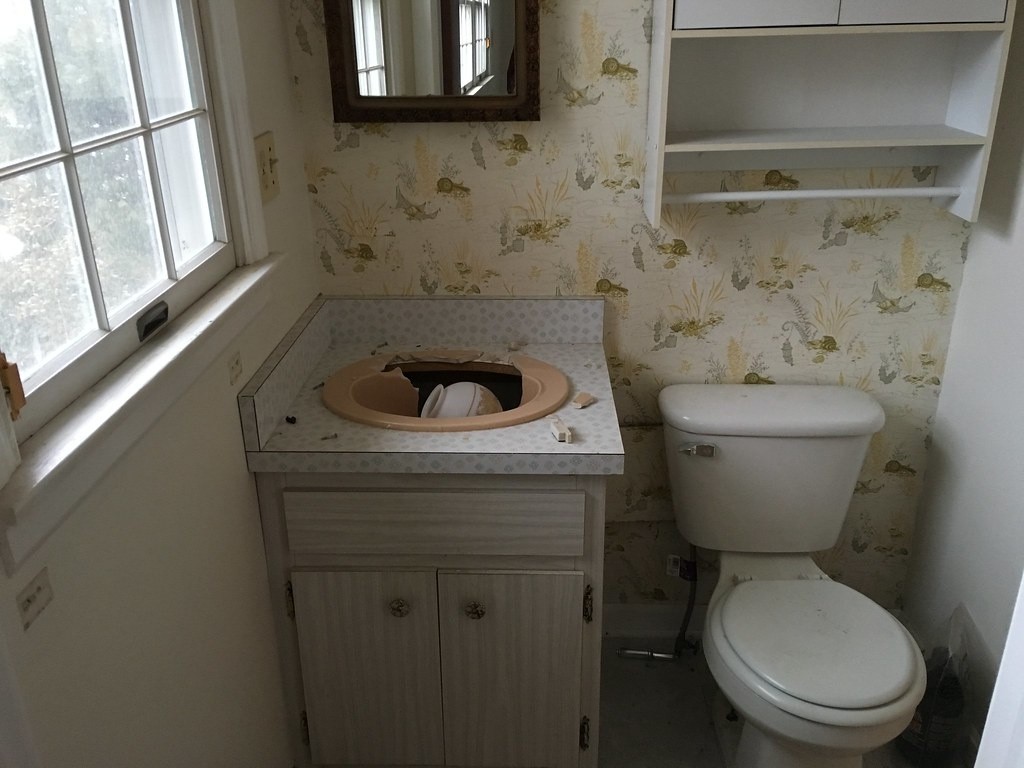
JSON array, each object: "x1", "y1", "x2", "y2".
[{"x1": 659, "y1": 383, "x2": 929, "y2": 768}]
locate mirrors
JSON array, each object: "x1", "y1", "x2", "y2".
[{"x1": 323, "y1": 0, "x2": 541, "y2": 124}]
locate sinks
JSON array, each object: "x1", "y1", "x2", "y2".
[{"x1": 322, "y1": 348, "x2": 571, "y2": 433}]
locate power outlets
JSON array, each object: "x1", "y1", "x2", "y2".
[
  {"x1": 254, "y1": 131, "x2": 280, "y2": 207},
  {"x1": 15, "y1": 566, "x2": 56, "y2": 634}
]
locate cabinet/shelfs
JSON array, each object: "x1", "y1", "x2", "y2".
[
  {"x1": 642, "y1": 0, "x2": 1015, "y2": 230},
  {"x1": 254, "y1": 471, "x2": 608, "y2": 768}
]
